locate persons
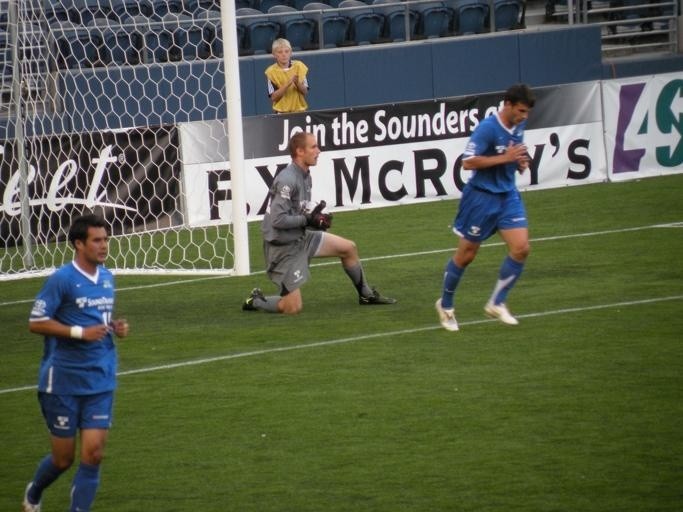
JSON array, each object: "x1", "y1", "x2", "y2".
[
  {"x1": 262, "y1": 38, "x2": 311, "y2": 114},
  {"x1": 18, "y1": 214, "x2": 130, "y2": 511},
  {"x1": 241, "y1": 131, "x2": 398, "y2": 316},
  {"x1": 434, "y1": 82, "x2": 537, "y2": 334}
]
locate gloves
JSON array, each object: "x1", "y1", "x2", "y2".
[{"x1": 306, "y1": 200, "x2": 334, "y2": 230}]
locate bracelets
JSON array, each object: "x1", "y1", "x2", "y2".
[{"x1": 69, "y1": 324, "x2": 83, "y2": 340}]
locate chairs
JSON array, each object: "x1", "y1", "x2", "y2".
[{"x1": 0, "y1": 0, "x2": 529, "y2": 104}]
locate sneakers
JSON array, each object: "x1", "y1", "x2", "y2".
[
  {"x1": 357, "y1": 288, "x2": 398, "y2": 304},
  {"x1": 435, "y1": 296, "x2": 459, "y2": 331},
  {"x1": 22, "y1": 481, "x2": 42, "y2": 511},
  {"x1": 483, "y1": 300, "x2": 520, "y2": 325},
  {"x1": 241, "y1": 288, "x2": 263, "y2": 311}
]
